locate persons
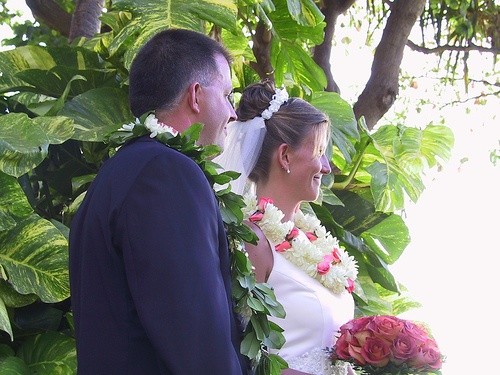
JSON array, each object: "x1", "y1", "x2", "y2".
[
  {"x1": 69, "y1": 26, "x2": 258, "y2": 375},
  {"x1": 228, "y1": 81, "x2": 358, "y2": 375}
]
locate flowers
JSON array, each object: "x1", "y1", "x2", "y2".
[
  {"x1": 114, "y1": 114, "x2": 186, "y2": 144},
  {"x1": 324, "y1": 311, "x2": 447, "y2": 375},
  {"x1": 239, "y1": 191, "x2": 360, "y2": 295}
]
